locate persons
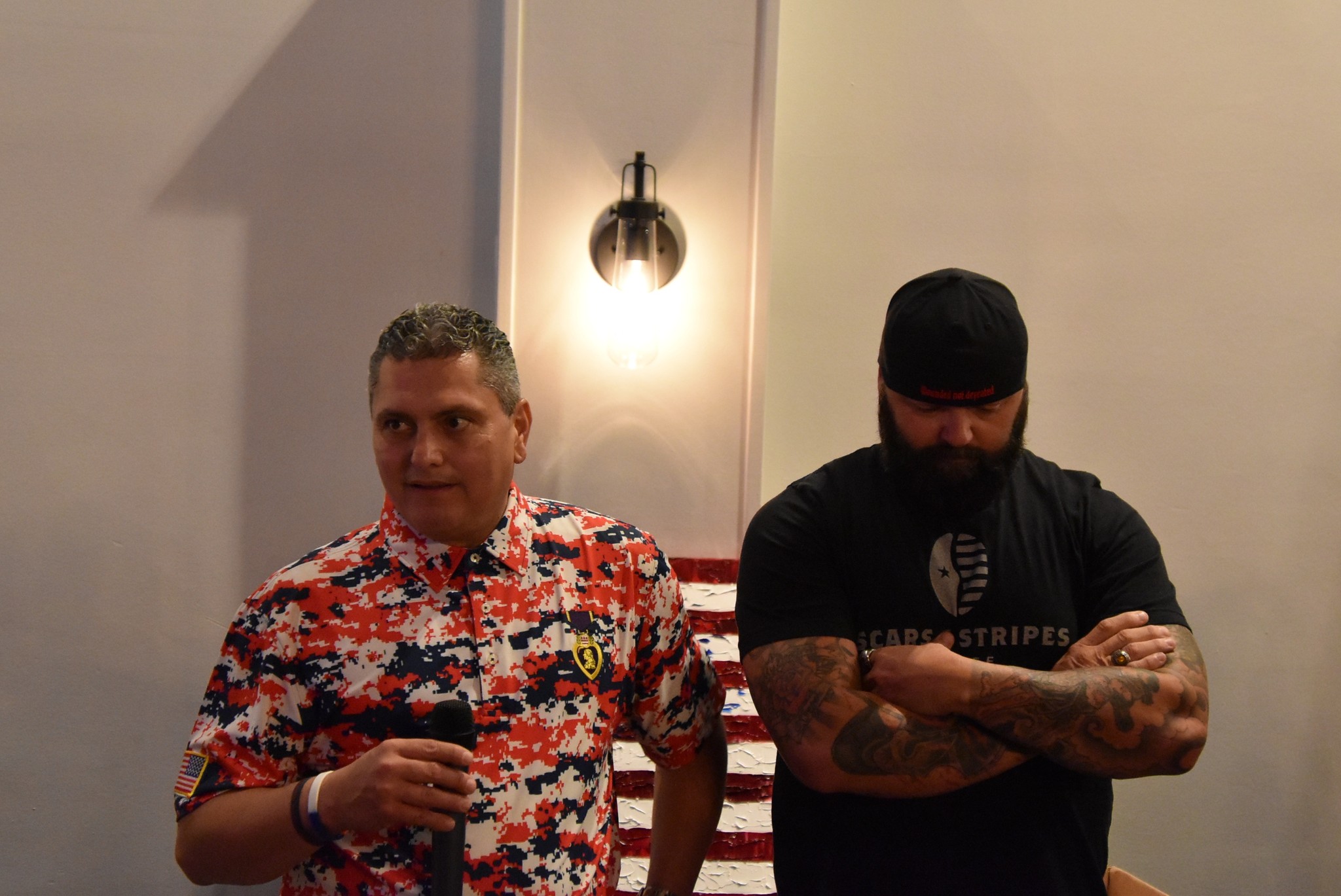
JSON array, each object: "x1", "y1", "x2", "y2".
[
  {"x1": 173, "y1": 304, "x2": 729, "y2": 896},
  {"x1": 737, "y1": 267, "x2": 1208, "y2": 896}
]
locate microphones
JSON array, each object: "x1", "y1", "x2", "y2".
[{"x1": 428, "y1": 698, "x2": 476, "y2": 896}]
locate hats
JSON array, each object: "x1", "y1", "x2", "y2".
[{"x1": 879, "y1": 270, "x2": 1031, "y2": 408}]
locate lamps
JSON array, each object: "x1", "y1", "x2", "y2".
[{"x1": 588, "y1": 151, "x2": 689, "y2": 329}]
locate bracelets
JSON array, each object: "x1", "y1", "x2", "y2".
[
  {"x1": 289, "y1": 778, "x2": 333, "y2": 847},
  {"x1": 307, "y1": 770, "x2": 348, "y2": 842}
]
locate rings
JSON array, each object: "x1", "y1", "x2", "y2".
[
  {"x1": 1112, "y1": 649, "x2": 1132, "y2": 666},
  {"x1": 859, "y1": 650, "x2": 876, "y2": 671}
]
